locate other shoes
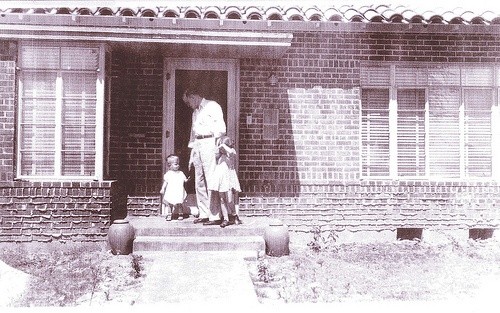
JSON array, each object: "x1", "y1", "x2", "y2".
[
  {"x1": 166, "y1": 215, "x2": 172, "y2": 221},
  {"x1": 178, "y1": 215, "x2": 184, "y2": 220},
  {"x1": 204, "y1": 220, "x2": 222, "y2": 225},
  {"x1": 194, "y1": 218, "x2": 208, "y2": 223},
  {"x1": 221, "y1": 219, "x2": 240, "y2": 228}
]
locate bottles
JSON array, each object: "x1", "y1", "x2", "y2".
[
  {"x1": 108, "y1": 219, "x2": 135, "y2": 255},
  {"x1": 264, "y1": 222, "x2": 289, "y2": 257}
]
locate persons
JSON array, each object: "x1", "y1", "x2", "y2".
[
  {"x1": 160, "y1": 154, "x2": 191, "y2": 221},
  {"x1": 182, "y1": 87, "x2": 226, "y2": 225},
  {"x1": 208, "y1": 136, "x2": 242, "y2": 227}
]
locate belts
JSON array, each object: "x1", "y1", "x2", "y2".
[{"x1": 193, "y1": 135, "x2": 213, "y2": 139}]
